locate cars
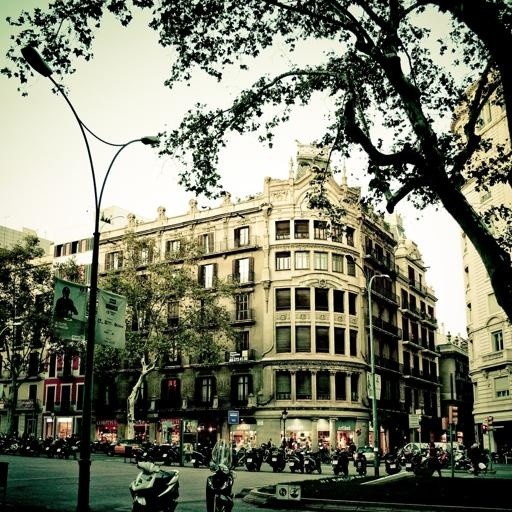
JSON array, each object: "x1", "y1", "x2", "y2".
[
  {"x1": 106, "y1": 439, "x2": 142, "y2": 456},
  {"x1": 352, "y1": 446, "x2": 378, "y2": 467},
  {"x1": 397, "y1": 442, "x2": 459, "y2": 460}
]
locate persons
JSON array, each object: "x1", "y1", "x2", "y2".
[{"x1": 288, "y1": 431, "x2": 482, "y2": 476}]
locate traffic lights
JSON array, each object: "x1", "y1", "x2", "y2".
[
  {"x1": 449, "y1": 406, "x2": 458, "y2": 424},
  {"x1": 484, "y1": 416, "x2": 493, "y2": 432}
]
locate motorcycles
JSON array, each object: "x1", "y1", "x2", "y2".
[
  {"x1": 198, "y1": 440, "x2": 247, "y2": 512},
  {"x1": 129, "y1": 447, "x2": 181, "y2": 512},
  {"x1": 262, "y1": 436, "x2": 491, "y2": 478},
  {"x1": 0, "y1": 429, "x2": 80, "y2": 460},
  {"x1": 90, "y1": 438, "x2": 263, "y2": 472}
]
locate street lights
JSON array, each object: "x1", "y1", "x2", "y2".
[
  {"x1": 16, "y1": 38, "x2": 164, "y2": 512},
  {"x1": 280, "y1": 408, "x2": 288, "y2": 443},
  {"x1": 346, "y1": 252, "x2": 395, "y2": 451}
]
